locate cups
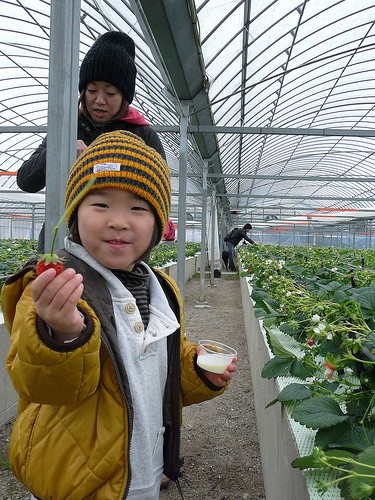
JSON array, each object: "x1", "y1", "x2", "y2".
[{"x1": 197, "y1": 340, "x2": 238, "y2": 374}]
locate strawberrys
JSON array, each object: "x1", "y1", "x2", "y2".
[
  {"x1": 308, "y1": 339, "x2": 313, "y2": 346},
  {"x1": 36, "y1": 254, "x2": 64, "y2": 278},
  {"x1": 323, "y1": 362, "x2": 335, "y2": 379}
]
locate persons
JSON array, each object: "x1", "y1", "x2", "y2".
[
  {"x1": 222, "y1": 223, "x2": 257, "y2": 272},
  {"x1": 17, "y1": 30, "x2": 167, "y2": 267},
  {"x1": 0, "y1": 129, "x2": 240, "y2": 500}
]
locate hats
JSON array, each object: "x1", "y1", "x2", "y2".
[
  {"x1": 64, "y1": 129, "x2": 172, "y2": 246},
  {"x1": 78, "y1": 31, "x2": 137, "y2": 104}
]
